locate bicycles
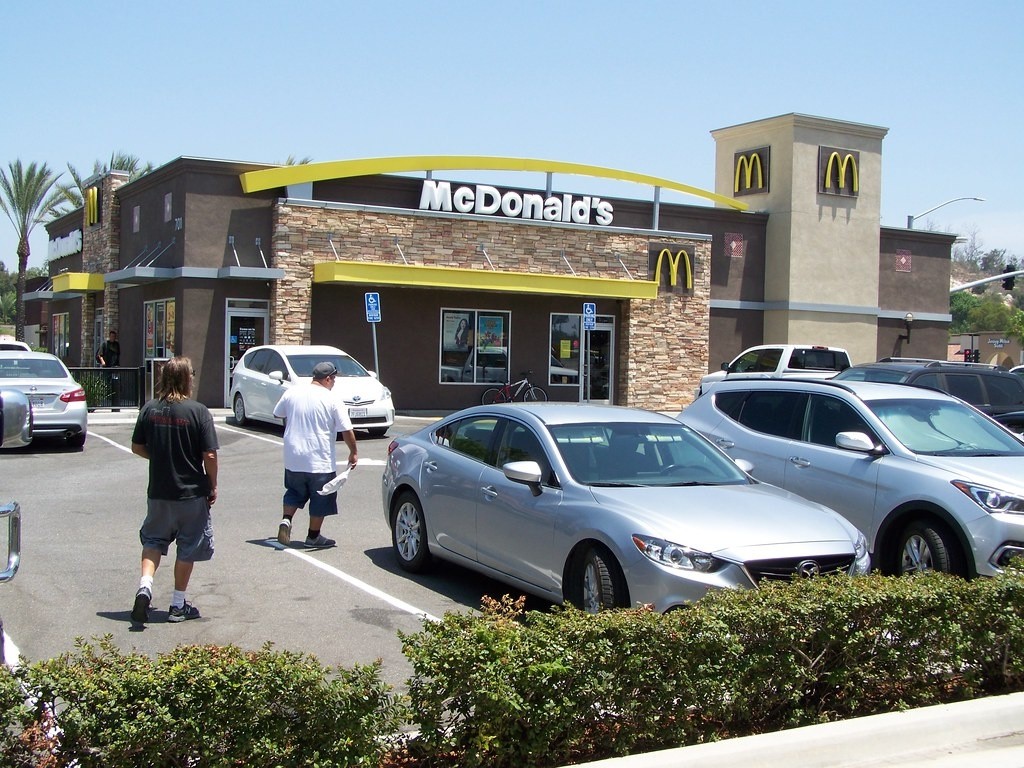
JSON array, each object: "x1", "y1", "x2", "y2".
[{"x1": 481, "y1": 368, "x2": 549, "y2": 406}]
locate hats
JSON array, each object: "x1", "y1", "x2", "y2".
[{"x1": 312, "y1": 361, "x2": 337, "y2": 380}]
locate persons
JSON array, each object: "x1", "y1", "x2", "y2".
[
  {"x1": 455, "y1": 318, "x2": 469, "y2": 346},
  {"x1": 131, "y1": 356, "x2": 220, "y2": 623},
  {"x1": 96, "y1": 331, "x2": 120, "y2": 397},
  {"x1": 272, "y1": 361, "x2": 357, "y2": 548}
]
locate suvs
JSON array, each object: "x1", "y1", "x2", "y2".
[
  {"x1": 695, "y1": 343, "x2": 853, "y2": 397},
  {"x1": 832, "y1": 361, "x2": 1023, "y2": 444},
  {"x1": 676, "y1": 377, "x2": 1024, "y2": 582}
]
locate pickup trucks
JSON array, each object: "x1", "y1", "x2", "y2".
[{"x1": 440, "y1": 347, "x2": 508, "y2": 384}]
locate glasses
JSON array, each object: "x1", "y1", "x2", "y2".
[{"x1": 191, "y1": 370, "x2": 196, "y2": 376}]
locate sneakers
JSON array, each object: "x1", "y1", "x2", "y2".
[
  {"x1": 277, "y1": 518, "x2": 293, "y2": 546},
  {"x1": 303, "y1": 533, "x2": 336, "y2": 548},
  {"x1": 166, "y1": 597, "x2": 200, "y2": 623},
  {"x1": 130, "y1": 587, "x2": 151, "y2": 624}
]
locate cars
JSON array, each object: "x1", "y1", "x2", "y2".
[
  {"x1": 551, "y1": 355, "x2": 578, "y2": 384},
  {"x1": 0, "y1": 339, "x2": 35, "y2": 376},
  {"x1": 230, "y1": 345, "x2": 395, "y2": 440},
  {"x1": 382, "y1": 403, "x2": 871, "y2": 629},
  {"x1": 0, "y1": 350, "x2": 88, "y2": 446}
]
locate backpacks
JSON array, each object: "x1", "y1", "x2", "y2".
[{"x1": 96, "y1": 340, "x2": 107, "y2": 364}]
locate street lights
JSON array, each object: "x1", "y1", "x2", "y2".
[{"x1": 906, "y1": 196, "x2": 987, "y2": 232}]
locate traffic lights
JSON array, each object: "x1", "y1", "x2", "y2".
[
  {"x1": 975, "y1": 349, "x2": 980, "y2": 363},
  {"x1": 964, "y1": 349, "x2": 971, "y2": 362},
  {"x1": 1001, "y1": 264, "x2": 1016, "y2": 290}
]
